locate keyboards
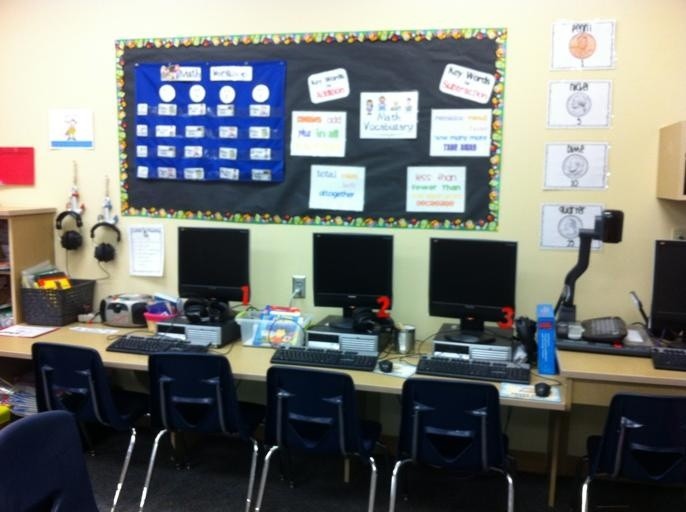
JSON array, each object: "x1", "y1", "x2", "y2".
[
  {"x1": 106, "y1": 334, "x2": 209, "y2": 355},
  {"x1": 652, "y1": 347, "x2": 685, "y2": 371},
  {"x1": 415, "y1": 354, "x2": 531, "y2": 385},
  {"x1": 271, "y1": 344, "x2": 378, "y2": 373}
]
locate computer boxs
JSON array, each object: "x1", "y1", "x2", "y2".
[
  {"x1": 432, "y1": 322, "x2": 514, "y2": 361},
  {"x1": 156, "y1": 312, "x2": 240, "y2": 347},
  {"x1": 305, "y1": 315, "x2": 394, "y2": 354}
]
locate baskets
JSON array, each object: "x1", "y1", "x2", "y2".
[{"x1": 19, "y1": 278, "x2": 96, "y2": 326}]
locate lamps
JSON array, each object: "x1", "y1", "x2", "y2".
[{"x1": 554, "y1": 208, "x2": 624, "y2": 332}]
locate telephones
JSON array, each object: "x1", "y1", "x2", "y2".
[{"x1": 582, "y1": 316, "x2": 627, "y2": 342}]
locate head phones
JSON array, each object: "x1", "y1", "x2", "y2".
[
  {"x1": 183, "y1": 296, "x2": 230, "y2": 323},
  {"x1": 516, "y1": 317, "x2": 536, "y2": 362},
  {"x1": 90, "y1": 222, "x2": 120, "y2": 262},
  {"x1": 55, "y1": 211, "x2": 82, "y2": 249},
  {"x1": 350, "y1": 306, "x2": 377, "y2": 330}
]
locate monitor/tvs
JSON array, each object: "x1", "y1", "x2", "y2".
[
  {"x1": 652, "y1": 239, "x2": 686, "y2": 341},
  {"x1": 429, "y1": 237, "x2": 518, "y2": 344},
  {"x1": 312, "y1": 232, "x2": 393, "y2": 330},
  {"x1": 177, "y1": 226, "x2": 250, "y2": 320}
]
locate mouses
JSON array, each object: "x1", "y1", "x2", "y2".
[
  {"x1": 380, "y1": 359, "x2": 393, "y2": 372},
  {"x1": 535, "y1": 383, "x2": 551, "y2": 396}
]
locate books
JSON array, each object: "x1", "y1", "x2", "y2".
[{"x1": 20, "y1": 261, "x2": 76, "y2": 308}]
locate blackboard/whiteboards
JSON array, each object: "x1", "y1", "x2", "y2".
[{"x1": 115, "y1": 26, "x2": 507, "y2": 232}]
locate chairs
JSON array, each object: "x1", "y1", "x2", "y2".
[
  {"x1": 138, "y1": 353, "x2": 257, "y2": 511},
  {"x1": 29, "y1": 343, "x2": 154, "y2": 512},
  {"x1": 253, "y1": 367, "x2": 382, "y2": 512},
  {"x1": 579, "y1": 392, "x2": 685, "y2": 512},
  {"x1": 0, "y1": 411, "x2": 97, "y2": 512},
  {"x1": 387, "y1": 379, "x2": 517, "y2": 512}
]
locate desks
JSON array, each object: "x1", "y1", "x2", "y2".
[
  {"x1": 1, "y1": 319, "x2": 565, "y2": 509},
  {"x1": 550, "y1": 341, "x2": 685, "y2": 508}
]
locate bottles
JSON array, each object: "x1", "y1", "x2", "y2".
[{"x1": 537, "y1": 303, "x2": 556, "y2": 376}]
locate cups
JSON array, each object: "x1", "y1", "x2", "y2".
[{"x1": 395, "y1": 325, "x2": 415, "y2": 355}]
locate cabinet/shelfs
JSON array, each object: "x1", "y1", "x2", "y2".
[
  {"x1": 0, "y1": 206, "x2": 56, "y2": 332},
  {"x1": 656, "y1": 122, "x2": 686, "y2": 201}
]
